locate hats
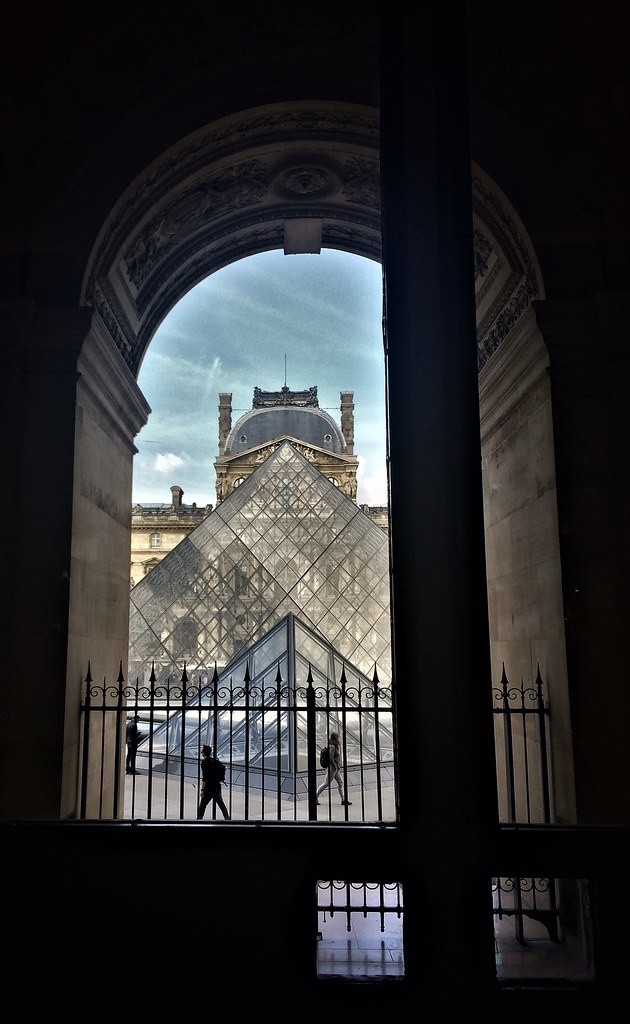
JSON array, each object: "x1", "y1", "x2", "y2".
[
  {"x1": 201, "y1": 745, "x2": 212, "y2": 755},
  {"x1": 330, "y1": 732, "x2": 340, "y2": 739}
]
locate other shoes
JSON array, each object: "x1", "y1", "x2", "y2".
[
  {"x1": 316, "y1": 800, "x2": 321, "y2": 805},
  {"x1": 341, "y1": 801, "x2": 352, "y2": 805},
  {"x1": 130, "y1": 771, "x2": 140, "y2": 775},
  {"x1": 126, "y1": 767, "x2": 131, "y2": 774}
]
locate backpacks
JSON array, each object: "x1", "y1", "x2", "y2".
[
  {"x1": 210, "y1": 758, "x2": 225, "y2": 782},
  {"x1": 320, "y1": 746, "x2": 332, "y2": 768}
]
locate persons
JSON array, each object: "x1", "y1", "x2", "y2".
[
  {"x1": 316, "y1": 733, "x2": 353, "y2": 805},
  {"x1": 126, "y1": 715, "x2": 141, "y2": 775},
  {"x1": 197, "y1": 744, "x2": 232, "y2": 820}
]
